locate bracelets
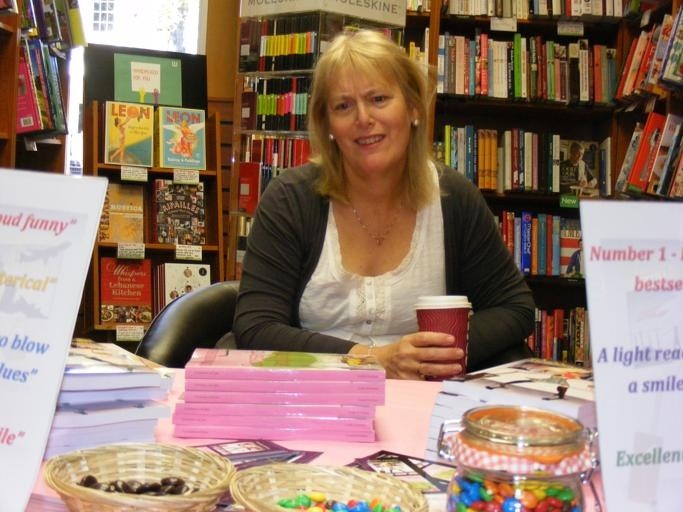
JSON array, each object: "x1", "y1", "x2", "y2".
[{"x1": 363, "y1": 342, "x2": 379, "y2": 357}]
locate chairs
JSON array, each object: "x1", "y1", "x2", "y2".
[{"x1": 126, "y1": 276, "x2": 247, "y2": 370}]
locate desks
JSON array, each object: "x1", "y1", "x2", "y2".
[{"x1": 29, "y1": 362, "x2": 605, "y2": 512}]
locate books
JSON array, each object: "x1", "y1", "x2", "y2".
[
  {"x1": 408, "y1": 0, "x2": 664, "y2": 18},
  {"x1": 495, "y1": 212, "x2": 585, "y2": 279},
  {"x1": 239, "y1": 135, "x2": 310, "y2": 213},
  {"x1": 615, "y1": 8, "x2": 682, "y2": 203},
  {"x1": 239, "y1": 24, "x2": 315, "y2": 71},
  {"x1": 104, "y1": 54, "x2": 206, "y2": 170},
  {"x1": 433, "y1": 124, "x2": 613, "y2": 199},
  {"x1": 235, "y1": 217, "x2": 256, "y2": 281},
  {"x1": 527, "y1": 308, "x2": 592, "y2": 370},
  {"x1": 403, "y1": 28, "x2": 621, "y2": 105},
  {"x1": 0, "y1": 0, "x2": 88, "y2": 156},
  {"x1": 241, "y1": 76, "x2": 312, "y2": 131},
  {"x1": 98, "y1": 180, "x2": 211, "y2": 326}
]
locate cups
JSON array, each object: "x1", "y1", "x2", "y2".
[{"x1": 412, "y1": 294, "x2": 474, "y2": 381}]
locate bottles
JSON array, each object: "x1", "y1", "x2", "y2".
[{"x1": 441, "y1": 407, "x2": 587, "y2": 512}]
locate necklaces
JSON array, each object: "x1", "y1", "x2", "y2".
[{"x1": 339, "y1": 179, "x2": 409, "y2": 250}]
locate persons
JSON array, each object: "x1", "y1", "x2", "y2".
[{"x1": 229, "y1": 23, "x2": 536, "y2": 383}]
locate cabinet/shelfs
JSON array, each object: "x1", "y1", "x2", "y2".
[
  {"x1": 84, "y1": 99, "x2": 227, "y2": 338},
  {"x1": 0, "y1": 11, "x2": 72, "y2": 173}
]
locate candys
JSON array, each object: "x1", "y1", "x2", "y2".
[
  {"x1": 447, "y1": 472, "x2": 581, "y2": 512},
  {"x1": 78, "y1": 475, "x2": 185, "y2": 498},
  {"x1": 279, "y1": 490, "x2": 404, "y2": 512}
]
